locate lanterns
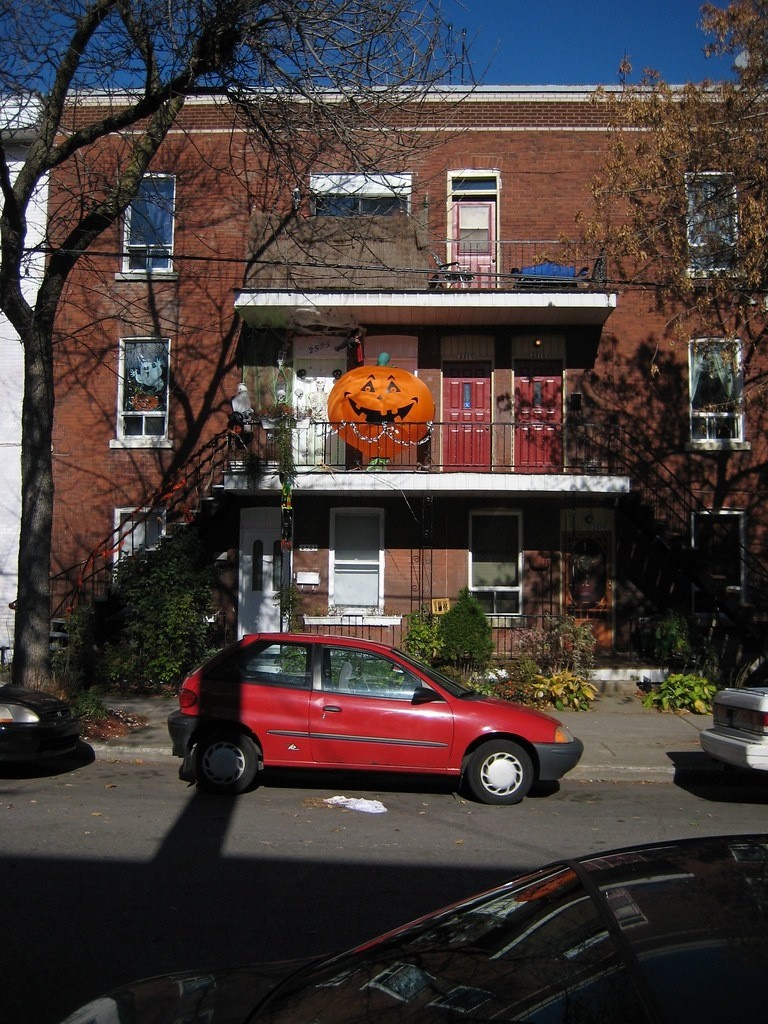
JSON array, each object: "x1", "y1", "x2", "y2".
[
  {"x1": 132, "y1": 392, "x2": 158, "y2": 411},
  {"x1": 328, "y1": 367, "x2": 434, "y2": 458}
]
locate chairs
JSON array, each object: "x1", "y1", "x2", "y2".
[
  {"x1": 338, "y1": 662, "x2": 352, "y2": 689},
  {"x1": 431, "y1": 252, "x2": 474, "y2": 289}
]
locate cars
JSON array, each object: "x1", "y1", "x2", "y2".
[
  {"x1": 0, "y1": 680, "x2": 86, "y2": 763},
  {"x1": 56, "y1": 832, "x2": 767, "y2": 1024},
  {"x1": 699, "y1": 685, "x2": 768, "y2": 774},
  {"x1": 166, "y1": 630, "x2": 585, "y2": 807}
]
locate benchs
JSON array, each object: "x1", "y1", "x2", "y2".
[{"x1": 511, "y1": 261, "x2": 589, "y2": 288}]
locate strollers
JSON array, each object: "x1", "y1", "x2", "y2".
[{"x1": 429, "y1": 251, "x2": 475, "y2": 289}]
